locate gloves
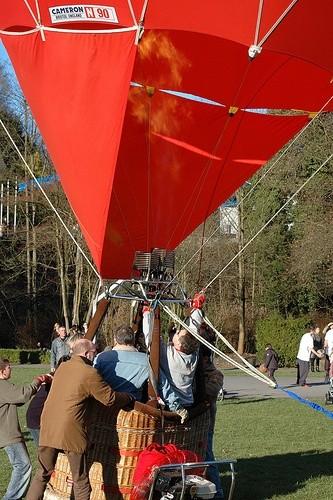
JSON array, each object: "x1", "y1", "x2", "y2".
[
  {"x1": 177, "y1": 407, "x2": 191, "y2": 425},
  {"x1": 142, "y1": 292, "x2": 155, "y2": 313},
  {"x1": 35, "y1": 374, "x2": 53, "y2": 383},
  {"x1": 192, "y1": 293, "x2": 204, "y2": 309},
  {"x1": 127, "y1": 392, "x2": 136, "y2": 407}
]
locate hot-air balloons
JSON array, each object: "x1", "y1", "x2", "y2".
[{"x1": 1, "y1": 0, "x2": 333, "y2": 500}]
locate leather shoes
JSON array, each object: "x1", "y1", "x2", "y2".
[{"x1": 302, "y1": 384, "x2": 311, "y2": 387}]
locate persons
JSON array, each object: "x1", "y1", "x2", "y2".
[
  {"x1": 140, "y1": 288, "x2": 206, "y2": 408},
  {"x1": 25, "y1": 338, "x2": 137, "y2": 500},
  {"x1": 264, "y1": 343, "x2": 278, "y2": 389},
  {"x1": 310, "y1": 326, "x2": 323, "y2": 372},
  {"x1": 26, "y1": 355, "x2": 71, "y2": 448},
  {"x1": 50, "y1": 326, "x2": 71, "y2": 373},
  {"x1": 325, "y1": 328, "x2": 333, "y2": 390},
  {"x1": 0, "y1": 358, "x2": 53, "y2": 500},
  {"x1": 51, "y1": 322, "x2": 61, "y2": 342},
  {"x1": 92, "y1": 324, "x2": 190, "y2": 425},
  {"x1": 198, "y1": 334, "x2": 225, "y2": 500},
  {"x1": 321, "y1": 323, "x2": 333, "y2": 383},
  {"x1": 296, "y1": 326, "x2": 317, "y2": 388},
  {"x1": 34, "y1": 341, "x2": 42, "y2": 349}
]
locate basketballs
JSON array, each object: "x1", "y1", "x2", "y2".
[
  {"x1": 317, "y1": 349, "x2": 325, "y2": 358},
  {"x1": 259, "y1": 363, "x2": 267, "y2": 373}
]
